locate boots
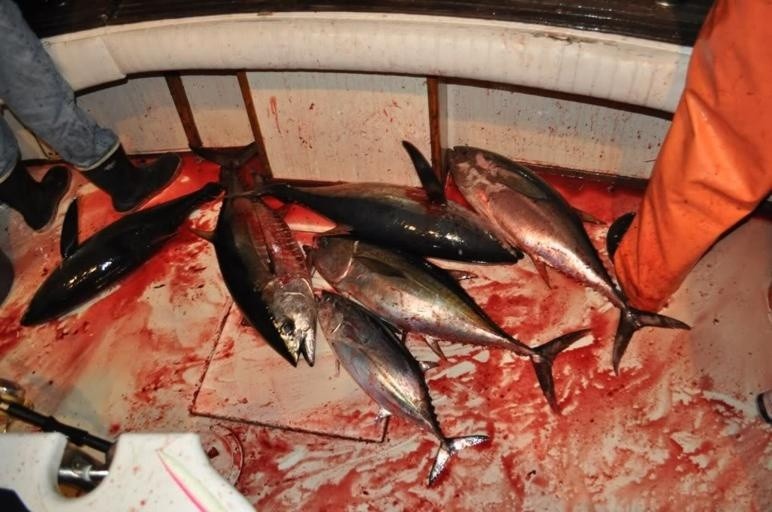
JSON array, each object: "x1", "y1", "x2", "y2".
[
  {"x1": 0, "y1": 153, "x2": 73, "y2": 234},
  {"x1": 73, "y1": 136, "x2": 182, "y2": 215}
]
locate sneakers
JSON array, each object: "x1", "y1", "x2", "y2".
[{"x1": 605, "y1": 212, "x2": 637, "y2": 264}]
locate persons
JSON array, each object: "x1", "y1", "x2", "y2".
[
  {"x1": 607, "y1": 0, "x2": 772, "y2": 312},
  {"x1": 0, "y1": 0, "x2": 182, "y2": 233}
]
